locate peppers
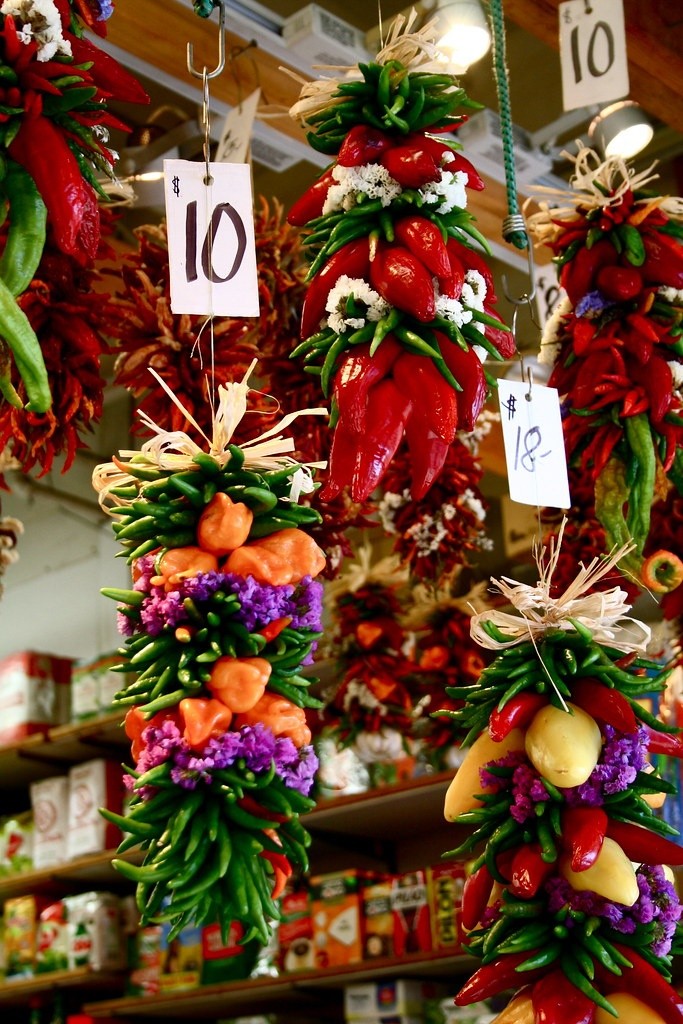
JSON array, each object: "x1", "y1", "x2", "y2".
[{"x1": 0, "y1": 0, "x2": 683, "y2": 1024}]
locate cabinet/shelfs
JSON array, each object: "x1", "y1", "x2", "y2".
[
  {"x1": 82, "y1": 741, "x2": 494, "y2": 1019},
  {"x1": 0, "y1": 688, "x2": 149, "y2": 1001}
]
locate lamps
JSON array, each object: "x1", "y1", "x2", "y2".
[
  {"x1": 286, "y1": 0, "x2": 495, "y2": 88},
  {"x1": 456, "y1": 93, "x2": 661, "y2": 215}
]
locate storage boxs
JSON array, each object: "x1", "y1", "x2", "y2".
[{"x1": 0, "y1": 635, "x2": 514, "y2": 1024}]
